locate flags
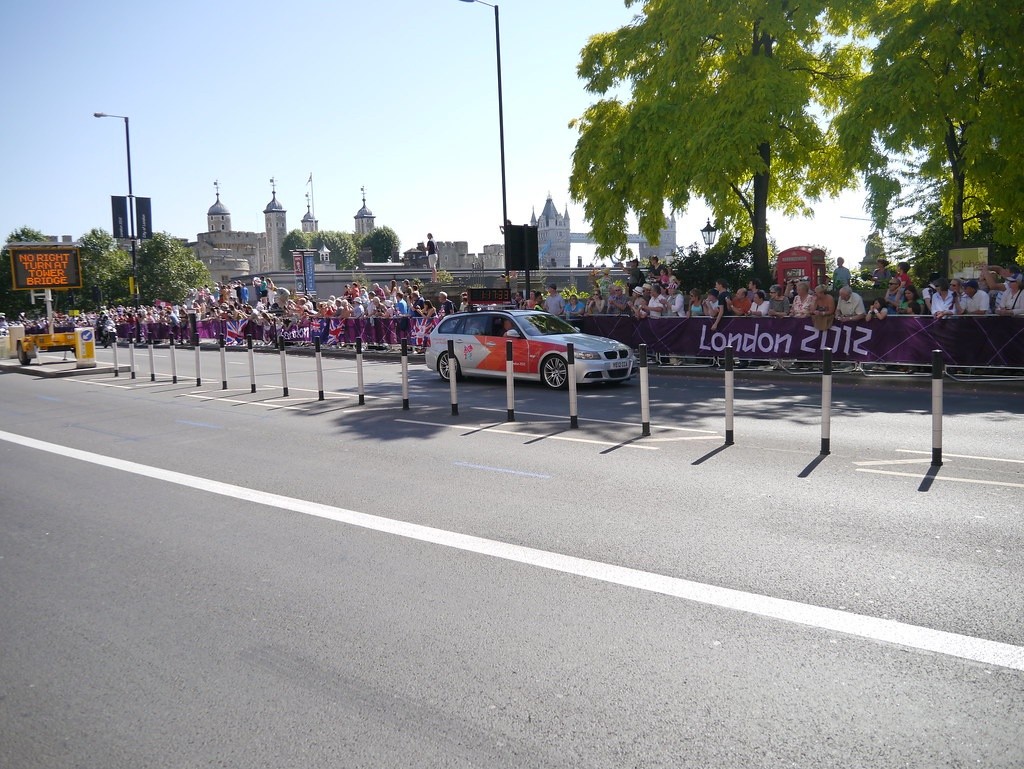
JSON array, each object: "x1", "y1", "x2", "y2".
[
  {"x1": 309, "y1": 316, "x2": 329, "y2": 344},
  {"x1": 224, "y1": 319, "x2": 251, "y2": 346},
  {"x1": 306, "y1": 176, "x2": 313, "y2": 184},
  {"x1": 410, "y1": 317, "x2": 441, "y2": 347},
  {"x1": 328, "y1": 316, "x2": 348, "y2": 345}
]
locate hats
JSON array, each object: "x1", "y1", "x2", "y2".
[
  {"x1": 602, "y1": 268, "x2": 610, "y2": 275},
  {"x1": 1005, "y1": 273, "x2": 1023, "y2": 284},
  {"x1": 642, "y1": 284, "x2": 653, "y2": 290},
  {"x1": 665, "y1": 283, "x2": 678, "y2": 289},
  {"x1": 961, "y1": 279, "x2": 977, "y2": 289},
  {"x1": 632, "y1": 286, "x2": 644, "y2": 295},
  {"x1": 710, "y1": 288, "x2": 719, "y2": 298}
]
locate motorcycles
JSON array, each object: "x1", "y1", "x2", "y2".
[{"x1": 98, "y1": 319, "x2": 118, "y2": 349}]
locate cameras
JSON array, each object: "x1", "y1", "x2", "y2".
[{"x1": 952, "y1": 291, "x2": 957, "y2": 297}]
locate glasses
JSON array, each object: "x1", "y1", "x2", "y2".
[
  {"x1": 593, "y1": 294, "x2": 600, "y2": 296},
  {"x1": 935, "y1": 285, "x2": 940, "y2": 287},
  {"x1": 615, "y1": 289, "x2": 621, "y2": 291},
  {"x1": 887, "y1": 283, "x2": 896, "y2": 285},
  {"x1": 949, "y1": 284, "x2": 957, "y2": 287}
]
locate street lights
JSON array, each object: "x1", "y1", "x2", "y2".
[
  {"x1": 93, "y1": 111, "x2": 142, "y2": 345},
  {"x1": 458, "y1": 0, "x2": 511, "y2": 303}
]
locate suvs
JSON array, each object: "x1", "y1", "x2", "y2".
[{"x1": 424, "y1": 310, "x2": 640, "y2": 389}]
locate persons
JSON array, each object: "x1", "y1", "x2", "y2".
[
  {"x1": 460, "y1": 292, "x2": 471, "y2": 310},
  {"x1": 513, "y1": 254, "x2": 1024, "y2": 372},
  {"x1": 465, "y1": 317, "x2": 484, "y2": 335},
  {"x1": 0, "y1": 272, "x2": 437, "y2": 349},
  {"x1": 461, "y1": 297, "x2": 472, "y2": 313},
  {"x1": 437, "y1": 289, "x2": 458, "y2": 316},
  {"x1": 497, "y1": 319, "x2": 522, "y2": 338},
  {"x1": 423, "y1": 233, "x2": 441, "y2": 283}
]
